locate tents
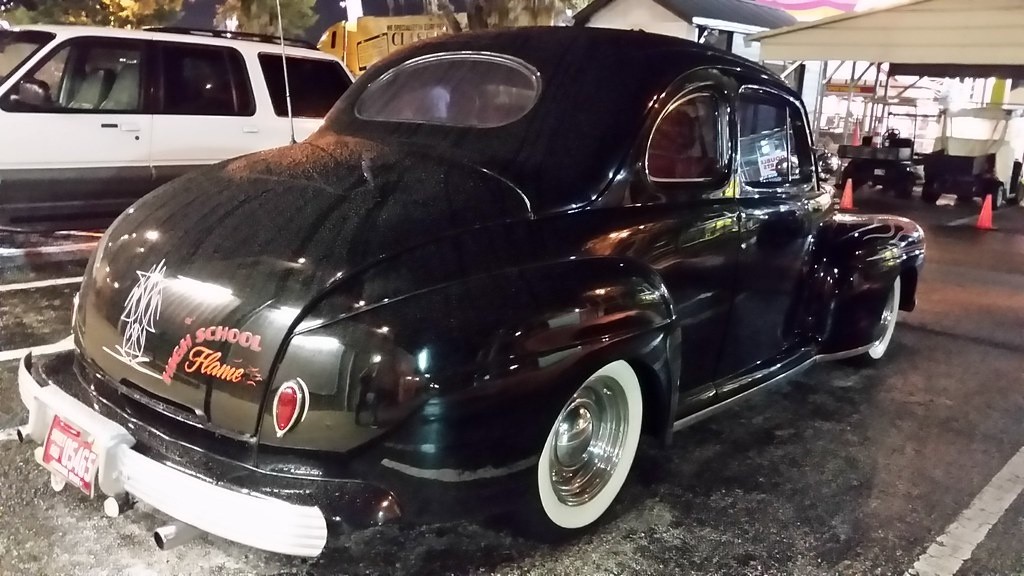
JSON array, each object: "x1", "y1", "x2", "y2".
[{"x1": 743, "y1": 0, "x2": 1024, "y2": 191}]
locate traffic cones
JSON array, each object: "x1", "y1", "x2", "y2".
[
  {"x1": 839, "y1": 179, "x2": 852, "y2": 208},
  {"x1": 977, "y1": 195, "x2": 992, "y2": 229},
  {"x1": 853, "y1": 128, "x2": 858, "y2": 146}
]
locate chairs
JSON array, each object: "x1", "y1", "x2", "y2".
[
  {"x1": 98, "y1": 63, "x2": 145, "y2": 109},
  {"x1": 67, "y1": 67, "x2": 116, "y2": 110}
]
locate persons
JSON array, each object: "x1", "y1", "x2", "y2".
[
  {"x1": 352, "y1": 357, "x2": 382, "y2": 430},
  {"x1": 419, "y1": 85, "x2": 450, "y2": 116}
]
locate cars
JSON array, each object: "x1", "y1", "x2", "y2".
[{"x1": 0, "y1": 25, "x2": 925, "y2": 561}]
